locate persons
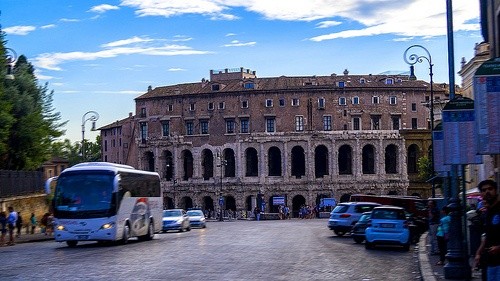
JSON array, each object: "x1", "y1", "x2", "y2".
[
  {"x1": 208, "y1": 204, "x2": 321, "y2": 222},
  {"x1": 0, "y1": 207, "x2": 54, "y2": 244},
  {"x1": 428, "y1": 179, "x2": 500, "y2": 281}
]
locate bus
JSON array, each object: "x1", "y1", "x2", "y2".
[
  {"x1": 350, "y1": 192, "x2": 429, "y2": 228},
  {"x1": 44, "y1": 162, "x2": 163, "y2": 247}
]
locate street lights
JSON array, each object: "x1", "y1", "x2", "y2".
[
  {"x1": 402, "y1": 44, "x2": 436, "y2": 197},
  {"x1": 81, "y1": 111, "x2": 100, "y2": 163},
  {"x1": 165, "y1": 155, "x2": 176, "y2": 209},
  {"x1": 213, "y1": 147, "x2": 222, "y2": 221}
]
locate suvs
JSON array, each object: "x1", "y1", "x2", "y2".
[{"x1": 327, "y1": 201, "x2": 382, "y2": 237}]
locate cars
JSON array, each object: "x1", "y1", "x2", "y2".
[
  {"x1": 186, "y1": 210, "x2": 206, "y2": 228},
  {"x1": 351, "y1": 206, "x2": 410, "y2": 250},
  {"x1": 162, "y1": 209, "x2": 191, "y2": 232}
]
